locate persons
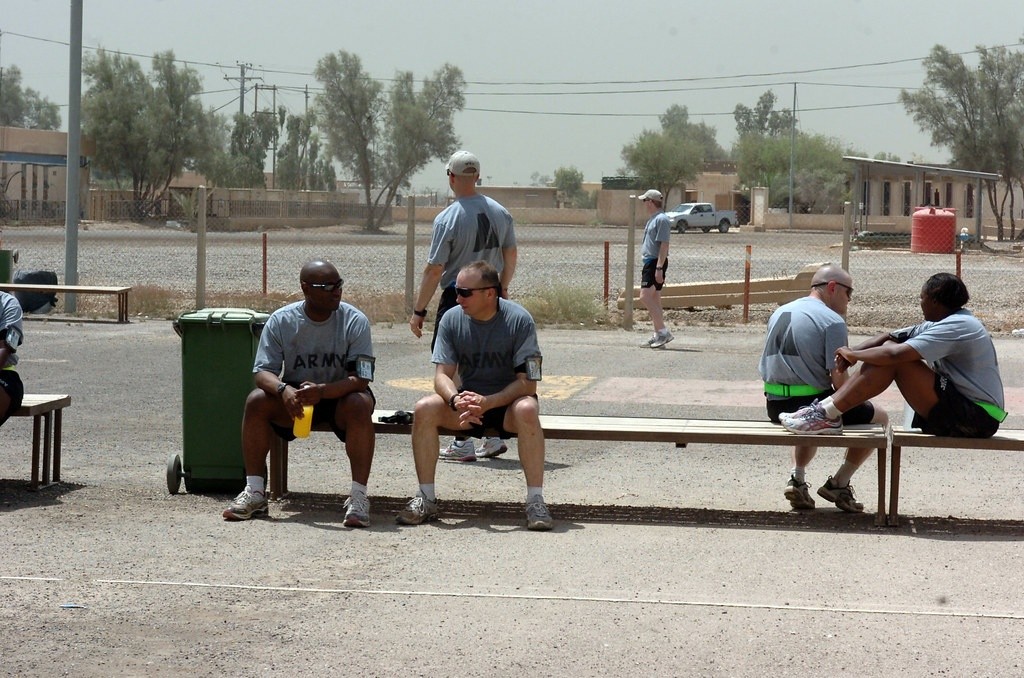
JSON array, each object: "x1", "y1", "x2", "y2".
[
  {"x1": 396, "y1": 260, "x2": 553, "y2": 529},
  {"x1": 222, "y1": 261, "x2": 376, "y2": 528},
  {"x1": 779, "y1": 273, "x2": 1008, "y2": 438},
  {"x1": 0, "y1": 292, "x2": 24, "y2": 426},
  {"x1": 409, "y1": 152, "x2": 517, "y2": 460},
  {"x1": 639, "y1": 189, "x2": 674, "y2": 348},
  {"x1": 760, "y1": 265, "x2": 888, "y2": 513}
]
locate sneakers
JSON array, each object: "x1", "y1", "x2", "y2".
[
  {"x1": 475, "y1": 439, "x2": 507, "y2": 457},
  {"x1": 525, "y1": 494, "x2": 553, "y2": 529},
  {"x1": 778, "y1": 398, "x2": 844, "y2": 435},
  {"x1": 817, "y1": 475, "x2": 863, "y2": 512},
  {"x1": 342, "y1": 491, "x2": 370, "y2": 527},
  {"x1": 651, "y1": 331, "x2": 674, "y2": 347},
  {"x1": 438, "y1": 442, "x2": 476, "y2": 461},
  {"x1": 784, "y1": 474, "x2": 815, "y2": 509},
  {"x1": 223, "y1": 485, "x2": 268, "y2": 520},
  {"x1": 640, "y1": 337, "x2": 665, "y2": 349},
  {"x1": 395, "y1": 491, "x2": 437, "y2": 524}
]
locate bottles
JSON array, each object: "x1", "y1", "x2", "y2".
[
  {"x1": 3, "y1": 354, "x2": 19, "y2": 370},
  {"x1": 293, "y1": 385, "x2": 313, "y2": 438}
]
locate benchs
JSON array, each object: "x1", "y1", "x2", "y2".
[
  {"x1": 12, "y1": 394, "x2": 71, "y2": 490},
  {"x1": 270, "y1": 410, "x2": 887, "y2": 526},
  {"x1": 888, "y1": 425, "x2": 1024, "y2": 531},
  {"x1": 0, "y1": 284, "x2": 132, "y2": 323}
]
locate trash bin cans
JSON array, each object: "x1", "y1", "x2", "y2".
[{"x1": 165, "y1": 306, "x2": 277, "y2": 495}]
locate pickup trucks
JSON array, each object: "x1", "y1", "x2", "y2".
[{"x1": 664, "y1": 202, "x2": 739, "y2": 233}]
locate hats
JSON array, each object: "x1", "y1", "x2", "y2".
[
  {"x1": 446, "y1": 151, "x2": 481, "y2": 176},
  {"x1": 638, "y1": 189, "x2": 663, "y2": 202}
]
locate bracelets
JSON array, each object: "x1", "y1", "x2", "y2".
[{"x1": 656, "y1": 267, "x2": 662, "y2": 270}]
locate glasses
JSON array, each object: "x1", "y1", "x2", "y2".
[
  {"x1": 446, "y1": 170, "x2": 453, "y2": 175},
  {"x1": 643, "y1": 199, "x2": 653, "y2": 202},
  {"x1": 307, "y1": 279, "x2": 344, "y2": 291},
  {"x1": 835, "y1": 282, "x2": 854, "y2": 296},
  {"x1": 454, "y1": 286, "x2": 495, "y2": 298}
]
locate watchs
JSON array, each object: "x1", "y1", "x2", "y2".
[
  {"x1": 278, "y1": 383, "x2": 289, "y2": 398},
  {"x1": 414, "y1": 308, "x2": 427, "y2": 317},
  {"x1": 449, "y1": 394, "x2": 459, "y2": 412}
]
